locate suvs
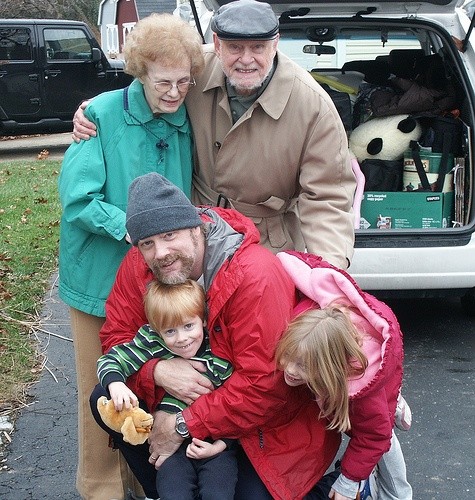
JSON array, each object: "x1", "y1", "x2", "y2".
[{"x1": 0, "y1": 17, "x2": 137, "y2": 136}]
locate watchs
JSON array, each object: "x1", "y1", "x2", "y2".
[{"x1": 175, "y1": 411, "x2": 190, "y2": 438}]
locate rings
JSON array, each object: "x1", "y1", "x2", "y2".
[{"x1": 150, "y1": 455, "x2": 156, "y2": 460}]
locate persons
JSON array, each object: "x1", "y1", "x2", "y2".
[
  {"x1": 58, "y1": 12, "x2": 204, "y2": 500},
  {"x1": 96, "y1": 279, "x2": 238, "y2": 500},
  {"x1": 90, "y1": 173, "x2": 344, "y2": 500},
  {"x1": 272, "y1": 250, "x2": 412, "y2": 500},
  {"x1": 71, "y1": 1, "x2": 358, "y2": 270}
]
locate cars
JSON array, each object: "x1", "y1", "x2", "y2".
[{"x1": 188, "y1": 1, "x2": 475, "y2": 288}]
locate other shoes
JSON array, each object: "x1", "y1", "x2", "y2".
[
  {"x1": 392, "y1": 394, "x2": 412, "y2": 431},
  {"x1": 358, "y1": 470, "x2": 379, "y2": 500}
]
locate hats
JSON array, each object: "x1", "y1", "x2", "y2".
[
  {"x1": 211, "y1": 0, "x2": 279, "y2": 41},
  {"x1": 125, "y1": 172, "x2": 203, "y2": 247}
]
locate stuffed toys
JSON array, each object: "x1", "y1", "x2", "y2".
[
  {"x1": 97, "y1": 395, "x2": 154, "y2": 446},
  {"x1": 349, "y1": 113, "x2": 423, "y2": 163}
]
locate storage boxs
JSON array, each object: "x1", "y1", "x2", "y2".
[{"x1": 360, "y1": 191, "x2": 454, "y2": 229}]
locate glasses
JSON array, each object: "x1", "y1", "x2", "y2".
[{"x1": 146, "y1": 72, "x2": 197, "y2": 93}]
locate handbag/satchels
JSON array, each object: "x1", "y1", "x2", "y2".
[
  {"x1": 410, "y1": 114, "x2": 464, "y2": 192},
  {"x1": 362, "y1": 159, "x2": 403, "y2": 192}
]
